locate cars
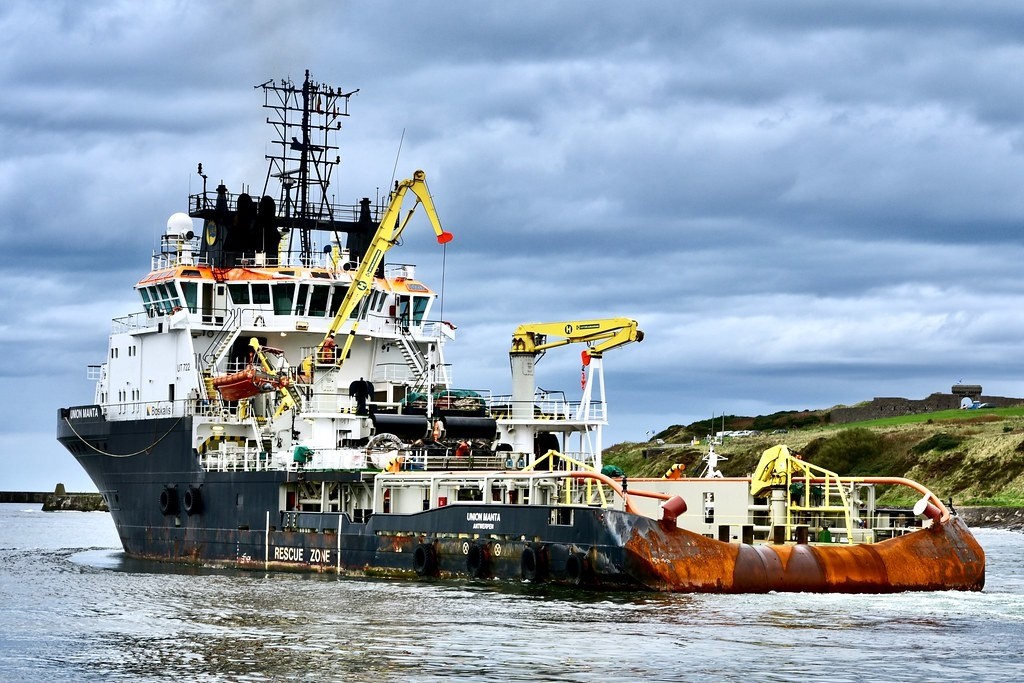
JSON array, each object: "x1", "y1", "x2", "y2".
[
  {"x1": 729, "y1": 431, "x2": 761, "y2": 438},
  {"x1": 656, "y1": 438, "x2": 665, "y2": 444},
  {"x1": 769, "y1": 429, "x2": 788, "y2": 435}
]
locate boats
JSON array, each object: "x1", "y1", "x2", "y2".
[{"x1": 55, "y1": 66, "x2": 684, "y2": 585}]
